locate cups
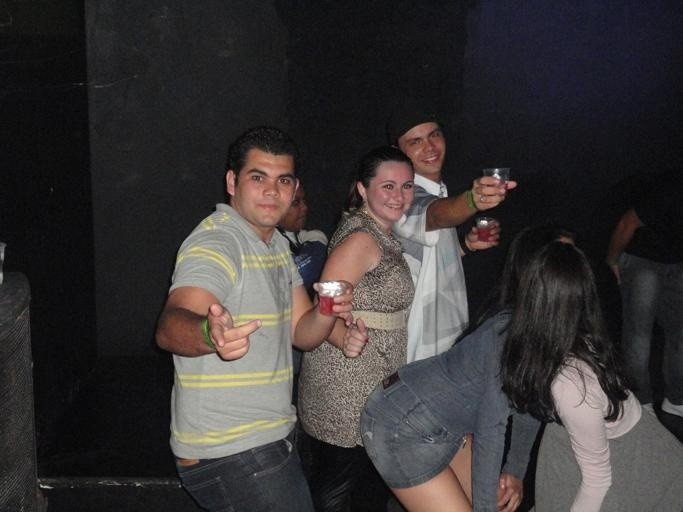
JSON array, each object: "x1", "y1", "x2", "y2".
[
  {"x1": 483, "y1": 167, "x2": 510, "y2": 196},
  {"x1": 476, "y1": 217, "x2": 496, "y2": 242},
  {"x1": 318, "y1": 281, "x2": 346, "y2": 316}
]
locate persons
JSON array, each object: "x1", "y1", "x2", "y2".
[
  {"x1": 500, "y1": 241, "x2": 683, "y2": 512},
  {"x1": 277, "y1": 186, "x2": 327, "y2": 406},
  {"x1": 387, "y1": 106, "x2": 517, "y2": 364},
  {"x1": 155, "y1": 126, "x2": 354, "y2": 512},
  {"x1": 600, "y1": 169, "x2": 683, "y2": 419},
  {"x1": 360, "y1": 225, "x2": 579, "y2": 512},
  {"x1": 298, "y1": 147, "x2": 415, "y2": 512}
]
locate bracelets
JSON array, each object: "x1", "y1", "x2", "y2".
[
  {"x1": 202, "y1": 318, "x2": 215, "y2": 351},
  {"x1": 467, "y1": 189, "x2": 480, "y2": 214},
  {"x1": 459, "y1": 232, "x2": 480, "y2": 258}
]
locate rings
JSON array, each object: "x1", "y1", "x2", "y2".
[{"x1": 480, "y1": 195, "x2": 483, "y2": 204}]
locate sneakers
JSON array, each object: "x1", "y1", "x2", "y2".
[
  {"x1": 660, "y1": 397, "x2": 683, "y2": 417},
  {"x1": 641, "y1": 403, "x2": 656, "y2": 417}
]
